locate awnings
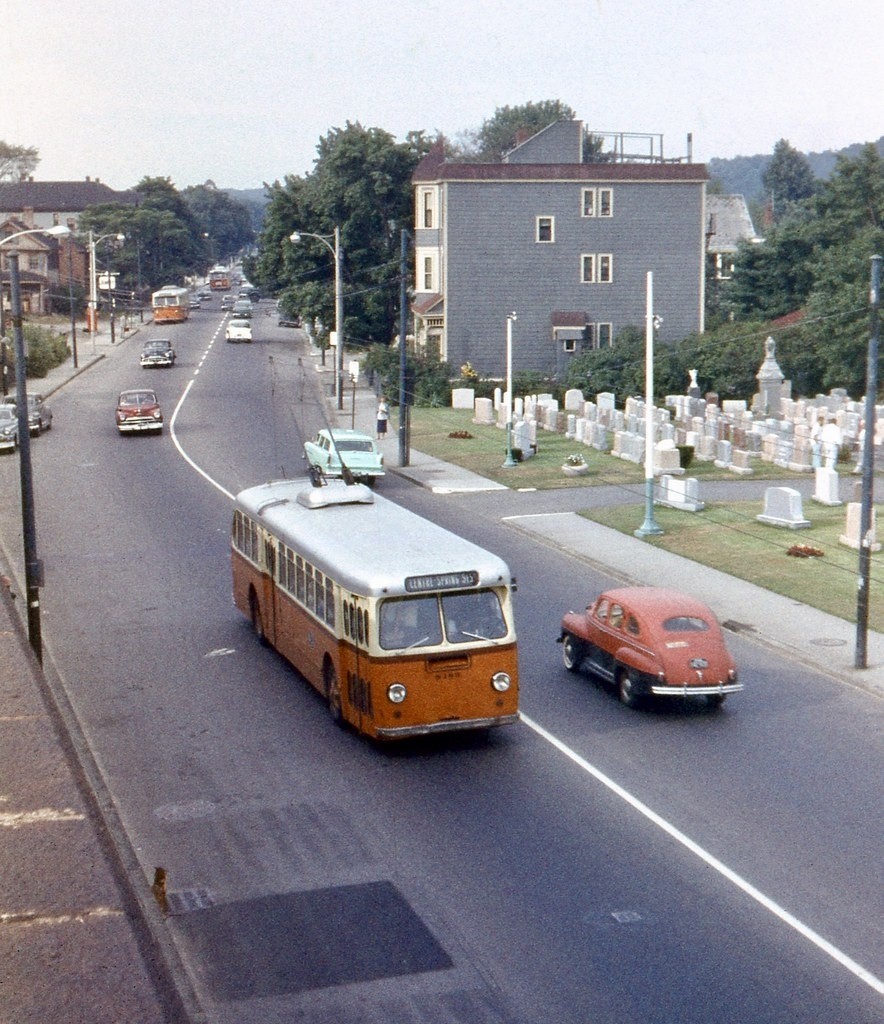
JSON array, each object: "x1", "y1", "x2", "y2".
[{"x1": 558, "y1": 329, "x2": 583, "y2": 340}]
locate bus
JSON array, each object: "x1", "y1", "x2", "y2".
[
  {"x1": 152, "y1": 285, "x2": 189, "y2": 324},
  {"x1": 208, "y1": 266, "x2": 231, "y2": 291},
  {"x1": 231, "y1": 478, "x2": 521, "y2": 742}
]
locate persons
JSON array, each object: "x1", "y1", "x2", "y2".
[
  {"x1": 287, "y1": 570, "x2": 333, "y2": 622},
  {"x1": 607, "y1": 605, "x2": 624, "y2": 628},
  {"x1": 380, "y1": 598, "x2": 420, "y2": 646},
  {"x1": 377, "y1": 395, "x2": 390, "y2": 440}
]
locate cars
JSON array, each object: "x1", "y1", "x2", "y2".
[
  {"x1": 230, "y1": 274, "x2": 242, "y2": 285},
  {"x1": 0, "y1": 403, "x2": 19, "y2": 454},
  {"x1": 238, "y1": 272, "x2": 247, "y2": 281},
  {"x1": 197, "y1": 287, "x2": 213, "y2": 301},
  {"x1": 556, "y1": 588, "x2": 743, "y2": 710},
  {"x1": 237, "y1": 288, "x2": 261, "y2": 303},
  {"x1": 3, "y1": 392, "x2": 53, "y2": 437},
  {"x1": 236, "y1": 294, "x2": 251, "y2": 300},
  {"x1": 115, "y1": 389, "x2": 163, "y2": 437},
  {"x1": 189, "y1": 294, "x2": 200, "y2": 309},
  {"x1": 221, "y1": 295, "x2": 235, "y2": 310},
  {"x1": 226, "y1": 320, "x2": 252, "y2": 343},
  {"x1": 278, "y1": 313, "x2": 303, "y2": 328},
  {"x1": 302, "y1": 429, "x2": 385, "y2": 487},
  {"x1": 276, "y1": 299, "x2": 283, "y2": 311},
  {"x1": 140, "y1": 339, "x2": 177, "y2": 369},
  {"x1": 233, "y1": 300, "x2": 252, "y2": 318}
]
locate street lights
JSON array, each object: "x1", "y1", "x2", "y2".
[
  {"x1": 90, "y1": 234, "x2": 125, "y2": 326},
  {"x1": 0, "y1": 224, "x2": 71, "y2": 244},
  {"x1": 289, "y1": 226, "x2": 344, "y2": 411}
]
